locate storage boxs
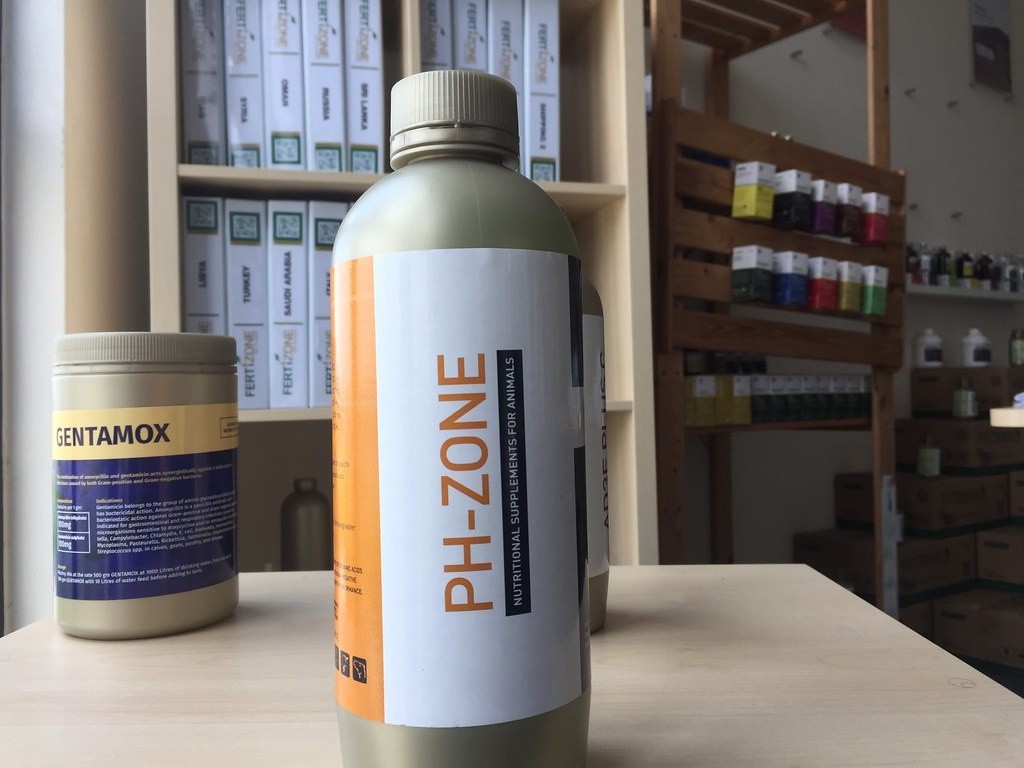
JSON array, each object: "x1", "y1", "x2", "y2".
[{"x1": 792, "y1": 367, "x2": 1024, "y2": 676}]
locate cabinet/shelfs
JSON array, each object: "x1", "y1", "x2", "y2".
[
  {"x1": 145, "y1": 0, "x2": 659, "y2": 565},
  {"x1": 645, "y1": 0, "x2": 899, "y2": 618}
]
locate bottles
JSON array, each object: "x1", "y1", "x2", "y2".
[
  {"x1": 913, "y1": 329, "x2": 944, "y2": 369},
  {"x1": 915, "y1": 433, "x2": 941, "y2": 479},
  {"x1": 951, "y1": 378, "x2": 979, "y2": 418},
  {"x1": 328, "y1": 68, "x2": 607, "y2": 768},
  {"x1": 906, "y1": 241, "x2": 1024, "y2": 293},
  {"x1": 1008, "y1": 329, "x2": 1024, "y2": 365},
  {"x1": 962, "y1": 328, "x2": 992, "y2": 367},
  {"x1": 49, "y1": 331, "x2": 241, "y2": 641}
]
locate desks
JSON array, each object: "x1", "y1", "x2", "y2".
[{"x1": 0, "y1": 564, "x2": 1024, "y2": 768}]
